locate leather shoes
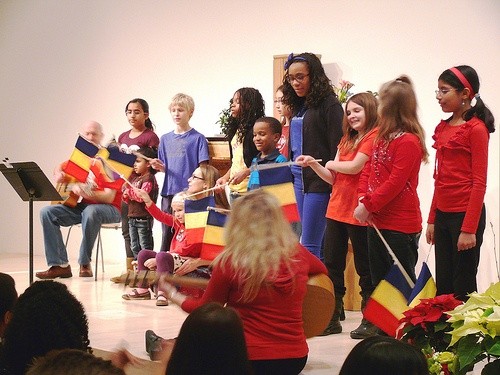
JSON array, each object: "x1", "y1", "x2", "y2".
[
  {"x1": 36, "y1": 264, "x2": 72, "y2": 279},
  {"x1": 79, "y1": 264, "x2": 93, "y2": 277}
]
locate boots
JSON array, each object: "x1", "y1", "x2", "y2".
[
  {"x1": 129, "y1": 260, "x2": 140, "y2": 288},
  {"x1": 110, "y1": 257, "x2": 133, "y2": 282}
]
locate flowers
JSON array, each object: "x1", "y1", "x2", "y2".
[
  {"x1": 332, "y1": 79, "x2": 357, "y2": 103},
  {"x1": 394, "y1": 281, "x2": 500, "y2": 375}
]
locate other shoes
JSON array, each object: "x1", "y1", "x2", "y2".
[
  {"x1": 315, "y1": 320, "x2": 342, "y2": 336},
  {"x1": 156, "y1": 294, "x2": 168, "y2": 306},
  {"x1": 146, "y1": 330, "x2": 167, "y2": 362},
  {"x1": 122, "y1": 288, "x2": 151, "y2": 300},
  {"x1": 350, "y1": 320, "x2": 388, "y2": 339}
]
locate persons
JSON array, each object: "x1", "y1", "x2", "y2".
[
  {"x1": 279, "y1": 52, "x2": 344, "y2": 260},
  {"x1": 122, "y1": 163, "x2": 230, "y2": 306},
  {"x1": 215, "y1": 83, "x2": 302, "y2": 242},
  {"x1": 110, "y1": 93, "x2": 210, "y2": 290},
  {"x1": 339, "y1": 336, "x2": 429, "y2": 375},
  {"x1": 31, "y1": 120, "x2": 121, "y2": 279},
  {"x1": 0, "y1": 275, "x2": 250, "y2": 375},
  {"x1": 156, "y1": 189, "x2": 329, "y2": 375},
  {"x1": 295, "y1": 75, "x2": 429, "y2": 339},
  {"x1": 426, "y1": 65, "x2": 495, "y2": 303}
]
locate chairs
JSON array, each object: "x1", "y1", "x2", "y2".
[{"x1": 65, "y1": 221, "x2": 122, "y2": 282}]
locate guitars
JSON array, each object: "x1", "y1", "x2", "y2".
[
  {"x1": 50, "y1": 173, "x2": 105, "y2": 207},
  {"x1": 110, "y1": 273, "x2": 336, "y2": 339}
]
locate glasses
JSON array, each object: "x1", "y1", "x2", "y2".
[
  {"x1": 435, "y1": 89, "x2": 463, "y2": 96},
  {"x1": 191, "y1": 173, "x2": 205, "y2": 180},
  {"x1": 286, "y1": 73, "x2": 310, "y2": 81},
  {"x1": 125, "y1": 111, "x2": 147, "y2": 116}
]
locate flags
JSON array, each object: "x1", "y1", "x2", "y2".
[
  {"x1": 258, "y1": 166, "x2": 301, "y2": 224},
  {"x1": 364, "y1": 260, "x2": 436, "y2": 341},
  {"x1": 63, "y1": 136, "x2": 136, "y2": 190},
  {"x1": 200, "y1": 210, "x2": 227, "y2": 261},
  {"x1": 185, "y1": 196, "x2": 215, "y2": 246}
]
